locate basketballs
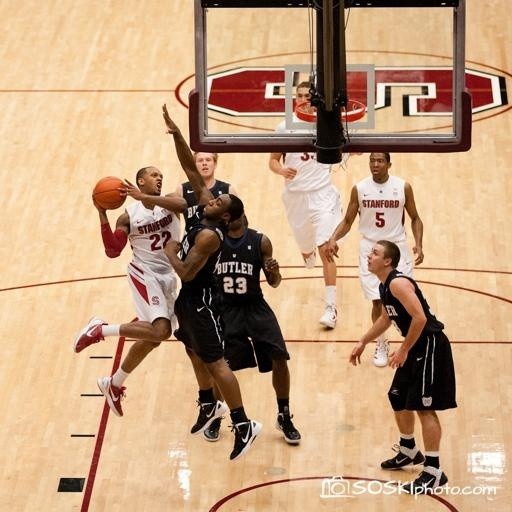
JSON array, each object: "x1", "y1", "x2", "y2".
[{"x1": 92, "y1": 176, "x2": 128, "y2": 210}]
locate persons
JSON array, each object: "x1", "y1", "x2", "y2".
[
  {"x1": 161, "y1": 102, "x2": 262, "y2": 461},
  {"x1": 323, "y1": 153, "x2": 425, "y2": 368},
  {"x1": 72, "y1": 166, "x2": 188, "y2": 417},
  {"x1": 348, "y1": 240, "x2": 458, "y2": 496},
  {"x1": 202, "y1": 214, "x2": 302, "y2": 447},
  {"x1": 268, "y1": 82, "x2": 346, "y2": 329},
  {"x1": 174, "y1": 150, "x2": 236, "y2": 233}
]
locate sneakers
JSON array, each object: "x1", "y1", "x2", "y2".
[
  {"x1": 320, "y1": 305, "x2": 338, "y2": 329},
  {"x1": 381, "y1": 450, "x2": 424, "y2": 470},
  {"x1": 401, "y1": 467, "x2": 447, "y2": 495},
  {"x1": 73, "y1": 317, "x2": 108, "y2": 353},
  {"x1": 204, "y1": 417, "x2": 221, "y2": 442},
  {"x1": 97, "y1": 376, "x2": 127, "y2": 416},
  {"x1": 274, "y1": 414, "x2": 302, "y2": 443},
  {"x1": 304, "y1": 249, "x2": 316, "y2": 267},
  {"x1": 191, "y1": 400, "x2": 229, "y2": 436},
  {"x1": 229, "y1": 420, "x2": 263, "y2": 461},
  {"x1": 374, "y1": 340, "x2": 389, "y2": 367}
]
